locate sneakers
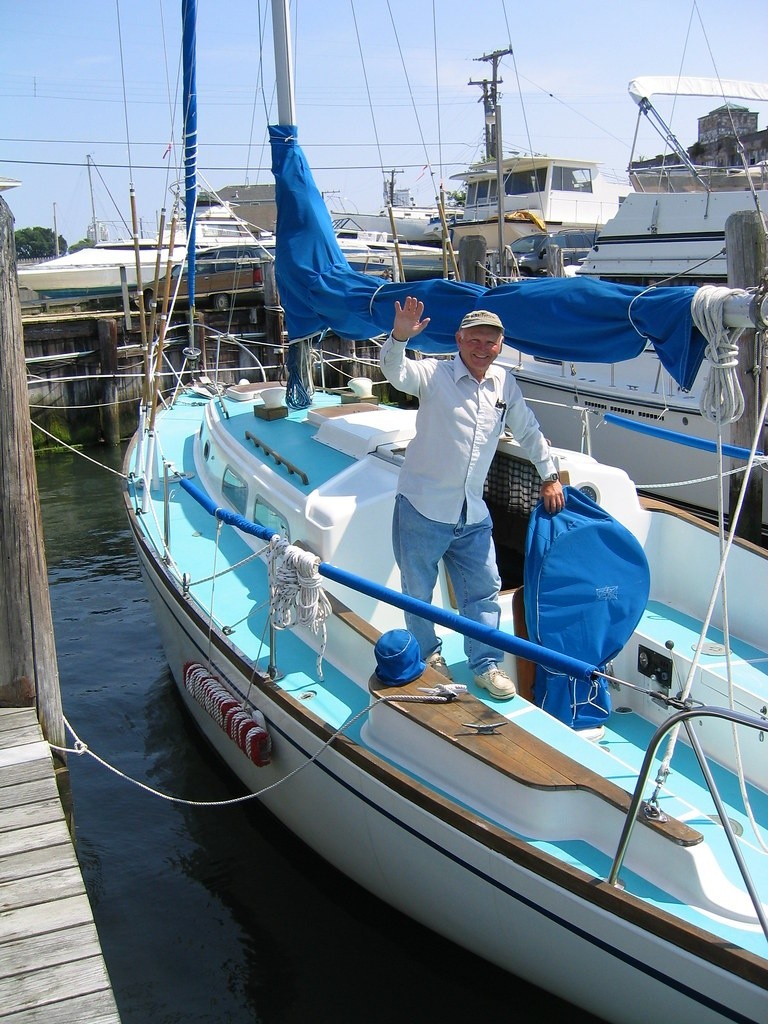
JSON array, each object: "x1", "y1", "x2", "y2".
[
  {"x1": 425, "y1": 653, "x2": 453, "y2": 682},
  {"x1": 475, "y1": 666, "x2": 516, "y2": 700}
]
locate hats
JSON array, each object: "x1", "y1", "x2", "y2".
[{"x1": 459, "y1": 310, "x2": 505, "y2": 333}]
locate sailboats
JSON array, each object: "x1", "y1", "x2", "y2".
[{"x1": 114, "y1": 1, "x2": 768, "y2": 1024}]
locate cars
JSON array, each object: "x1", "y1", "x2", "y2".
[{"x1": 134, "y1": 242, "x2": 279, "y2": 313}]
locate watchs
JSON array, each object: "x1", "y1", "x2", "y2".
[{"x1": 544, "y1": 473, "x2": 560, "y2": 482}]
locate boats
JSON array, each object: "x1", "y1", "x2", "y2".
[{"x1": 15, "y1": 0, "x2": 768, "y2": 545}]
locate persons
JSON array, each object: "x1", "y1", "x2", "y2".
[{"x1": 378, "y1": 297, "x2": 566, "y2": 699}]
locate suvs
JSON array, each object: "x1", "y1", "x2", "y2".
[{"x1": 501, "y1": 228, "x2": 602, "y2": 277}]
